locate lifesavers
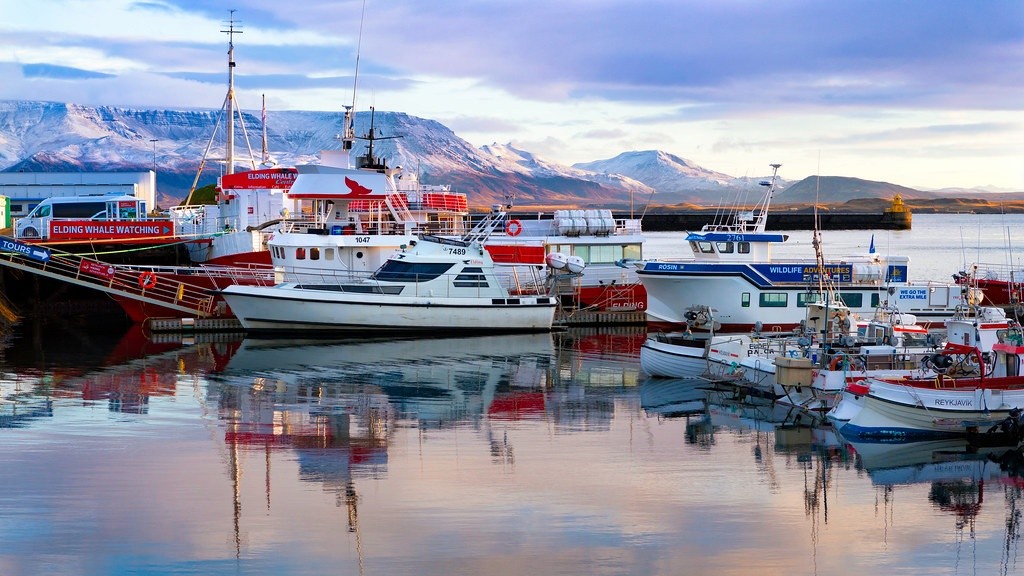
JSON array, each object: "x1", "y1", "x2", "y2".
[
  {"x1": 829, "y1": 353, "x2": 857, "y2": 373},
  {"x1": 504, "y1": 219, "x2": 523, "y2": 238},
  {"x1": 137, "y1": 270, "x2": 158, "y2": 289}
]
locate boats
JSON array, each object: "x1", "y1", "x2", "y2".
[
  {"x1": 202, "y1": 204, "x2": 586, "y2": 335},
  {"x1": 639, "y1": 159, "x2": 947, "y2": 420},
  {"x1": 953, "y1": 203, "x2": 1023, "y2": 307},
  {"x1": 832, "y1": 429, "x2": 1024, "y2": 576},
  {"x1": 168, "y1": 8, "x2": 655, "y2": 311},
  {"x1": 633, "y1": 162, "x2": 970, "y2": 332},
  {"x1": 826, "y1": 205, "x2": 1024, "y2": 439}
]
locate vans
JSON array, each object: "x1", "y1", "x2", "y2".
[{"x1": 15, "y1": 195, "x2": 136, "y2": 239}]
